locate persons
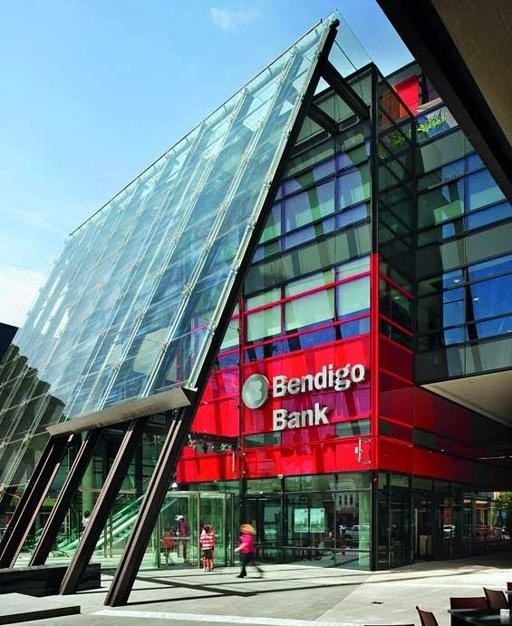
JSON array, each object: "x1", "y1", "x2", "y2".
[
  {"x1": 82, "y1": 511, "x2": 91, "y2": 530},
  {"x1": 199, "y1": 526, "x2": 215, "y2": 572},
  {"x1": 234, "y1": 523, "x2": 264, "y2": 578},
  {"x1": 164, "y1": 529, "x2": 173, "y2": 564},
  {"x1": 201, "y1": 523, "x2": 214, "y2": 570},
  {"x1": 176, "y1": 514, "x2": 190, "y2": 564}
]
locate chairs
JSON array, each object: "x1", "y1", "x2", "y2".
[{"x1": 363, "y1": 581, "x2": 512, "y2": 626}]
